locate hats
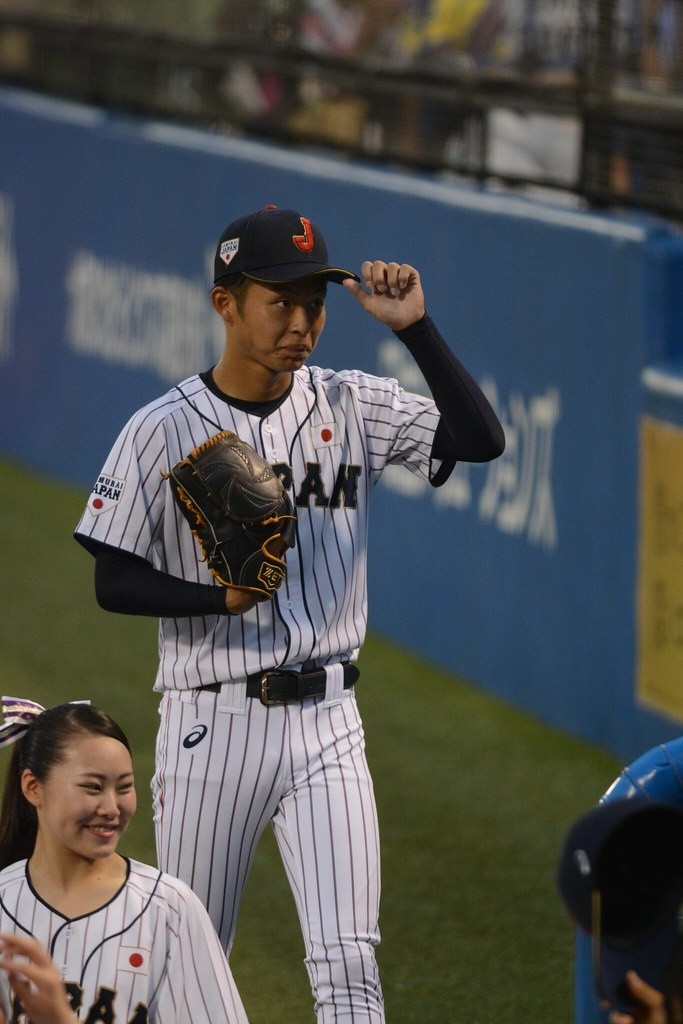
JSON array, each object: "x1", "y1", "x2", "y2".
[
  {"x1": 558, "y1": 797, "x2": 683, "y2": 1013},
  {"x1": 214, "y1": 204, "x2": 361, "y2": 286}
]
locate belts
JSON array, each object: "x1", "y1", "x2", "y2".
[{"x1": 191, "y1": 661, "x2": 360, "y2": 706}]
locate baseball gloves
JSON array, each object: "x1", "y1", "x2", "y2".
[{"x1": 156, "y1": 429, "x2": 300, "y2": 602}]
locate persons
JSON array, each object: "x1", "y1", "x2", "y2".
[
  {"x1": 601, "y1": 971, "x2": 683, "y2": 1024},
  {"x1": 0, "y1": 692, "x2": 249, "y2": 1024},
  {"x1": 209, "y1": 0, "x2": 588, "y2": 207},
  {"x1": 73, "y1": 201, "x2": 506, "y2": 1024}
]
